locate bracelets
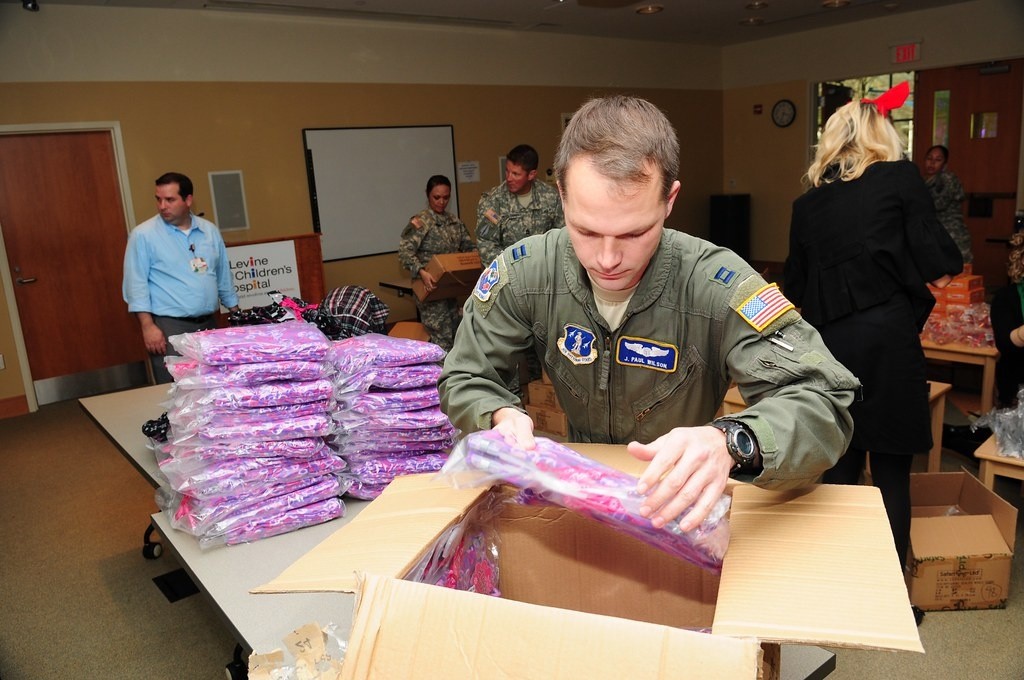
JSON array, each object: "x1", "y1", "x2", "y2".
[{"x1": 1017, "y1": 327, "x2": 1024, "y2": 343}]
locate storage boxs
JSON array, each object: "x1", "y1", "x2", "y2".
[
  {"x1": 412, "y1": 250, "x2": 483, "y2": 303},
  {"x1": 901, "y1": 464, "x2": 1019, "y2": 611},
  {"x1": 250, "y1": 441, "x2": 927, "y2": 680}
]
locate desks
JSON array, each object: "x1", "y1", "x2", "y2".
[
  {"x1": 721, "y1": 376, "x2": 951, "y2": 486},
  {"x1": 974, "y1": 434, "x2": 1024, "y2": 492},
  {"x1": 922, "y1": 302, "x2": 999, "y2": 417},
  {"x1": 149, "y1": 499, "x2": 376, "y2": 680},
  {"x1": 379, "y1": 279, "x2": 420, "y2": 322},
  {"x1": 78, "y1": 382, "x2": 169, "y2": 492}
]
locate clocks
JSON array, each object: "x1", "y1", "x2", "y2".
[{"x1": 771, "y1": 100, "x2": 796, "y2": 128}]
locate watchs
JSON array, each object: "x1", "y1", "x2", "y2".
[{"x1": 712, "y1": 421, "x2": 756, "y2": 471}]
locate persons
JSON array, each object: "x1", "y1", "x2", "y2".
[
  {"x1": 474, "y1": 144, "x2": 567, "y2": 268},
  {"x1": 990, "y1": 228, "x2": 1024, "y2": 408},
  {"x1": 783, "y1": 81, "x2": 963, "y2": 626},
  {"x1": 436, "y1": 95, "x2": 860, "y2": 533},
  {"x1": 122, "y1": 172, "x2": 239, "y2": 385},
  {"x1": 922, "y1": 144, "x2": 974, "y2": 264},
  {"x1": 399, "y1": 174, "x2": 478, "y2": 370}
]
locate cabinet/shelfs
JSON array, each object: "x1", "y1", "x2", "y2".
[{"x1": 710, "y1": 194, "x2": 750, "y2": 260}]
